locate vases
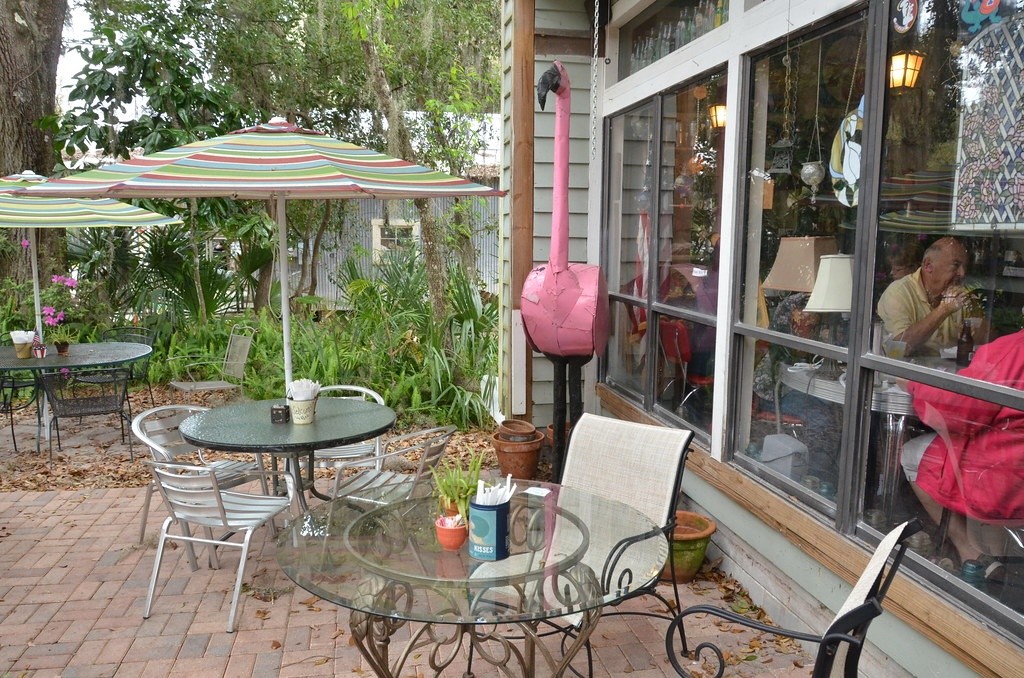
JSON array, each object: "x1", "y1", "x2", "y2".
[
  {"x1": 662, "y1": 510, "x2": 715, "y2": 584},
  {"x1": 498, "y1": 419, "x2": 535, "y2": 442},
  {"x1": 546, "y1": 420, "x2": 573, "y2": 453},
  {"x1": 491, "y1": 432, "x2": 544, "y2": 478}
]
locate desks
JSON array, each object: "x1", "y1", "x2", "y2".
[
  {"x1": 182, "y1": 398, "x2": 396, "y2": 578},
  {"x1": 278, "y1": 478, "x2": 668, "y2": 678},
  {"x1": 0, "y1": 343, "x2": 153, "y2": 462}
]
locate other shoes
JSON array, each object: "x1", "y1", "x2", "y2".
[{"x1": 962, "y1": 553, "x2": 1007, "y2": 602}]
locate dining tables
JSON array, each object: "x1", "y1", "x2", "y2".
[{"x1": 778, "y1": 359, "x2": 967, "y2": 536}]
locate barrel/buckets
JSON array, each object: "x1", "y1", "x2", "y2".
[
  {"x1": 468, "y1": 495, "x2": 511, "y2": 562},
  {"x1": 288, "y1": 396, "x2": 317, "y2": 425},
  {"x1": 15, "y1": 343, "x2": 33, "y2": 359},
  {"x1": 491, "y1": 419, "x2": 717, "y2": 584}
]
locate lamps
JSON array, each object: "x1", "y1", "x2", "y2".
[
  {"x1": 710, "y1": 104, "x2": 726, "y2": 131},
  {"x1": 887, "y1": 49, "x2": 925, "y2": 93},
  {"x1": 759, "y1": 236, "x2": 854, "y2": 382}
]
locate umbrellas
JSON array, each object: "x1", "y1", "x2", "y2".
[
  {"x1": 10, "y1": 117, "x2": 507, "y2": 510},
  {"x1": 0, "y1": 170, "x2": 184, "y2": 440}
]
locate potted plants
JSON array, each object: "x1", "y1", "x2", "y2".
[
  {"x1": 52, "y1": 327, "x2": 74, "y2": 355},
  {"x1": 428, "y1": 446, "x2": 484, "y2": 515}
]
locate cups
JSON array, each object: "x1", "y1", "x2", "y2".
[
  {"x1": 887, "y1": 340, "x2": 906, "y2": 361},
  {"x1": 435, "y1": 516, "x2": 469, "y2": 552}
]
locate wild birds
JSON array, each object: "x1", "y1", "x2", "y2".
[{"x1": 520, "y1": 61, "x2": 610, "y2": 483}]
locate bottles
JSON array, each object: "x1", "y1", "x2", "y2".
[{"x1": 956, "y1": 319, "x2": 974, "y2": 370}]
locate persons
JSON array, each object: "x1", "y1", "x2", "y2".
[
  {"x1": 672, "y1": 237, "x2": 720, "y2": 427},
  {"x1": 877, "y1": 236, "x2": 998, "y2": 498},
  {"x1": 896, "y1": 293, "x2": 1024, "y2": 600},
  {"x1": 752, "y1": 292, "x2": 840, "y2": 496}
]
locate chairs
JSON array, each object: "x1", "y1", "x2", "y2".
[
  {"x1": 654, "y1": 319, "x2": 713, "y2": 420},
  {"x1": 0, "y1": 324, "x2": 458, "y2": 631},
  {"x1": 936, "y1": 508, "x2": 1024, "y2": 614},
  {"x1": 464, "y1": 413, "x2": 921, "y2": 678}
]
